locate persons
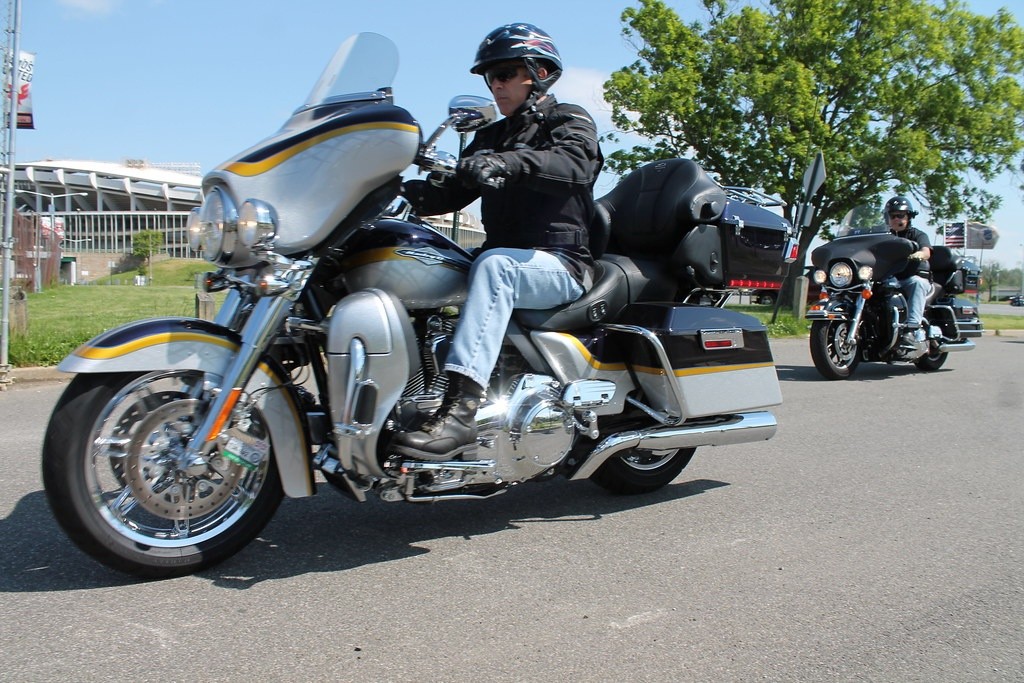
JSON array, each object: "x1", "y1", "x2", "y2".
[
  {"x1": 390, "y1": 25, "x2": 603, "y2": 463},
  {"x1": 883, "y1": 197, "x2": 933, "y2": 349}
]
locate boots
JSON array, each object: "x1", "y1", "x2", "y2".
[{"x1": 389, "y1": 374, "x2": 484, "y2": 462}]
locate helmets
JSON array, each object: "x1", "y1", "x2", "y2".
[
  {"x1": 470, "y1": 22, "x2": 563, "y2": 76},
  {"x1": 883, "y1": 197, "x2": 913, "y2": 212}
]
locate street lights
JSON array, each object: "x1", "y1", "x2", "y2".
[
  {"x1": 63, "y1": 238, "x2": 91, "y2": 262},
  {"x1": 13, "y1": 189, "x2": 88, "y2": 250}
]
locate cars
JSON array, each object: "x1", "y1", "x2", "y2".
[{"x1": 750, "y1": 266, "x2": 820, "y2": 306}]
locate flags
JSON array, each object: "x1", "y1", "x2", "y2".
[{"x1": 945, "y1": 220, "x2": 999, "y2": 249}]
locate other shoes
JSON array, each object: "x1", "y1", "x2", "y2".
[{"x1": 900, "y1": 328, "x2": 917, "y2": 347}]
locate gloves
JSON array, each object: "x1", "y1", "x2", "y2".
[{"x1": 456, "y1": 153, "x2": 508, "y2": 188}]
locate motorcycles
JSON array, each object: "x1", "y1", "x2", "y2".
[
  {"x1": 42, "y1": 29, "x2": 801, "y2": 580},
  {"x1": 803, "y1": 198, "x2": 999, "y2": 378}
]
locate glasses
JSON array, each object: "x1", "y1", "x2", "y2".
[
  {"x1": 485, "y1": 62, "x2": 526, "y2": 85},
  {"x1": 887, "y1": 213, "x2": 906, "y2": 219}
]
